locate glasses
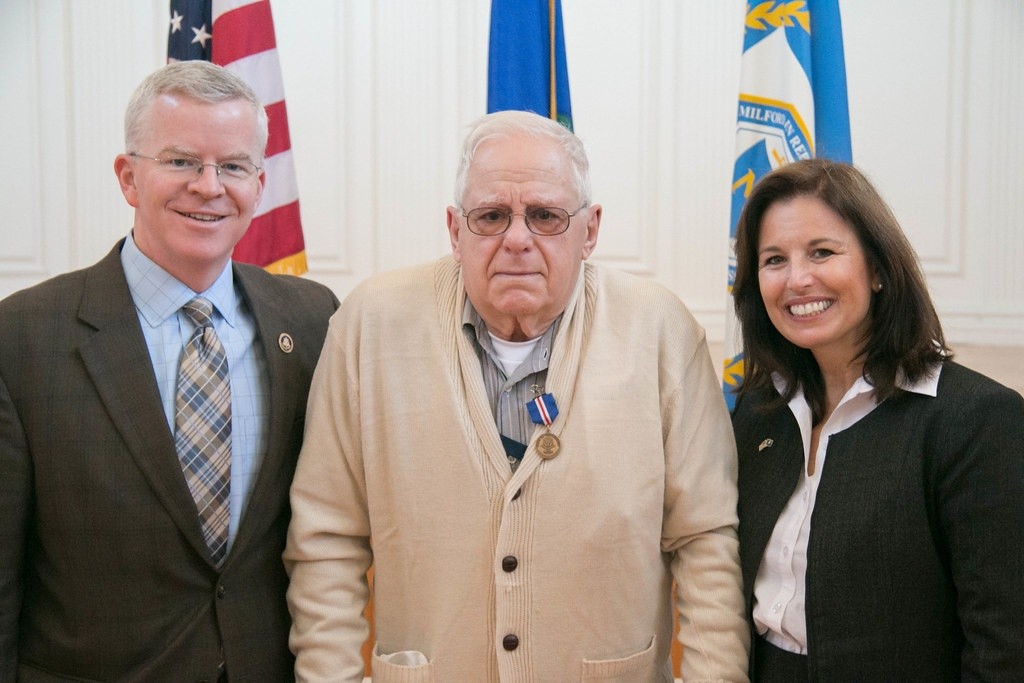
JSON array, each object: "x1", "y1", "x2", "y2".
[
  {"x1": 459, "y1": 203, "x2": 585, "y2": 236},
  {"x1": 130, "y1": 153, "x2": 262, "y2": 187}
]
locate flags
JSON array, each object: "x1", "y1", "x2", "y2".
[
  {"x1": 486, "y1": 0, "x2": 574, "y2": 133},
  {"x1": 721, "y1": 0, "x2": 853, "y2": 416},
  {"x1": 166, "y1": 0, "x2": 310, "y2": 279}
]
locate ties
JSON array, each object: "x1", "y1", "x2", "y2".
[{"x1": 175, "y1": 297, "x2": 233, "y2": 576}]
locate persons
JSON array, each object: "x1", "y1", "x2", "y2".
[
  {"x1": 283, "y1": 111, "x2": 749, "y2": 683},
  {"x1": 730, "y1": 157, "x2": 1024, "y2": 683},
  {"x1": 0, "y1": 60, "x2": 337, "y2": 683}
]
